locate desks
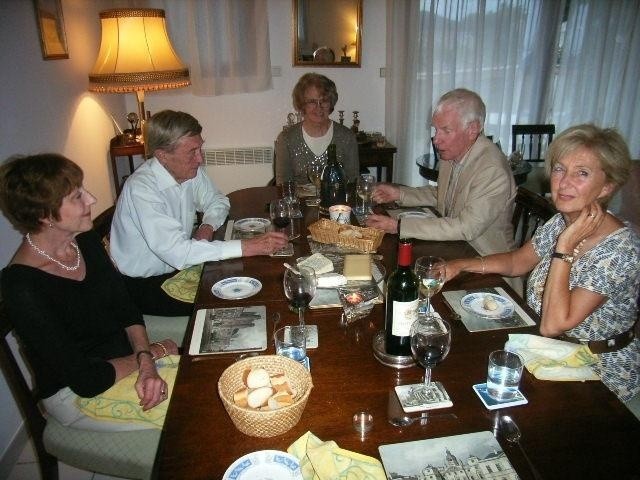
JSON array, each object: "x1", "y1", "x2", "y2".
[
  {"x1": 350, "y1": 130, "x2": 398, "y2": 185},
  {"x1": 415, "y1": 150, "x2": 533, "y2": 190},
  {"x1": 108, "y1": 125, "x2": 146, "y2": 204}
]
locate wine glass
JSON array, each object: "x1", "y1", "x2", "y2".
[
  {"x1": 408, "y1": 317, "x2": 452, "y2": 403},
  {"x1": 307, "y1": 163, "x2": 323, "y2": 206},
  {"x1": 268, "y1": 181, "x2": 302, "y2": 258},
  {"x1": 284, "y1": 266, "x2": 316, "y2": 335},
  {"x1": 354, "y1": 174, "x2": 376, "y2": 221},
  {"x1": 413, "y1": 255, "x2": 447, "y2": 325}
]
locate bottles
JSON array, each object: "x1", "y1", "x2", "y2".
[
  {"x1": 380, "y1": 236, "x2": 422, "y2": 356},
  {"x1": 321, "y1": 144, "x2": 346, "y2": 206}
]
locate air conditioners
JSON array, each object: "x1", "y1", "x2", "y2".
[{"x1": 195, "y1": 144, "x2": 276, "y2": 201}]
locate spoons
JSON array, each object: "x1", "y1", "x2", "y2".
[
  {"x1": 389, "y1": 415, "x2": 459, "y2": 426},
  {"x1": 500, "y1": 416, "x2": 543, "y2": 479},
  {"x1": 191, "y1": 350, "x2": 260, "y2": 362}
]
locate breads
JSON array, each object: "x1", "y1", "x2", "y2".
[
  {"x1": 233, "y1": 368, "x2": 297, "y2": 411},
  {"x1": 338, "y1": 225, "x2": 364, "y2": 238}
]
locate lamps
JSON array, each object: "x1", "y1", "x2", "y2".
[{"x1": 87, "y1": 6, "x2": 191, "y2": 146}]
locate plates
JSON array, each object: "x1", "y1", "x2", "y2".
[
  {"x1": 222, "y1": 448, "x2": 304, "y2": 480},
  {"x1": 461, "y1": 291, "x2": 514, "y2": 322},
  {"x1": 212, "y1": 276, "x2": 262, "y2": 300},
  {"x1": 396, "y1": 211, "x2": 437, "y2": 221},
  {"x1": 373, "y1": 328, "x2": 413, "y2": 369},
  {"x1": 314, "y1": 46, "x2": 336, "y2": 63},
  {"x1": 315, "y1": 256, "x2": 387, "y2": 290},
  {"x1": 233, "y1": 218, "x2": 271, "y2": 231}
]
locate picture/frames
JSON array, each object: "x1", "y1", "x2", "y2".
[{"x1": 289, "y1": 0, "x2": 364, "y2": 70}]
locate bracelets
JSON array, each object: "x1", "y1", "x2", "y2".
[
  {"x1": 472, "y1": 256, "x2": 488, "y2": 274},
  {"x1": 553, "y1": 252, "x2": 575, "y2": 267},
  {"x1": 151, "y1": 339, "x2": 171, "y2": 359},
  {"x1": 398, "y1": 219, "x2": 403, "y2": 237},
  {"x1": 134, "y1": 349, "x2": 155, "y2": 362}
]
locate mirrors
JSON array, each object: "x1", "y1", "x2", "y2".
[{"x1": 32, "y1": 0, "x2": 72, "y2": 63}]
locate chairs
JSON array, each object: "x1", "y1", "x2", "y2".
[
  {"x1": 510, "y1": 121, "x2": 556, "y2": 199},
  {"x1": 87, "y1": 203, "x2": 196, "y2": 357},
  {"x1": 498, "y1": 182, "x2": 559, "y2": 308},
  {"x1": 0, "y1": 265, "x2": 166, "y2": 480}
]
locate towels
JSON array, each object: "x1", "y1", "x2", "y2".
[
  {"x1": 72, "y1": 350, "x2": 182, "y2": 431},
  {"x1": 286, "y1": 430, "x2": 387, "y2": 480},
  {"x1": 503, "y1": 331, "x2": 603, "y2": 384},
  {"x1": 159, "y1": 261, "x2": 207, "y2": 307}
]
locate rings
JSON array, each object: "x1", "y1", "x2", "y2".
[
  {"x1": 587, "y1": 212, "x2": 598, "y2": 219},
  {"x1": 160, "y1": 390, "x2": 166, "y2": 397}
]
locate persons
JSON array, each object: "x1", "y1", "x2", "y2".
[
  {"x1": 432, "y1": 120, "x2": 640, "y2": 422},
  {"x1": 274, "y1": 73, "x2": 362, "y2": 186},
  {"x1": 107, "y1": 107, "x2": 289, "y2": 318},
  {"x1": 0, "y1": 154, "x2": 186, "y2": 433},
  {"x1": 365, "y1": 88, "x2": 525, "y2": 300},
  {"x1": 310, "y1": 46, "x2": 335, "y2": 62}
]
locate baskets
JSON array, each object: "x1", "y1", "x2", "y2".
[
  {"x1": 217, "y1": 355, "x2": 314, "y2": 438},
  {"x1": 306, "y1": 216, "x2": 387, "y2": 254}
]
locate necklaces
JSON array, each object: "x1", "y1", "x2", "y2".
[
  {"x1": 26, "y1": 229, "x2": 81, "y2": 274},
  {"x1": 574, "y1": 238, "x2": 588, "y2": 258}
]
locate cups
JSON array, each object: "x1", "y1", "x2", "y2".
[
  {"x1": 328, "y1": 204, "x2": 352, "y2": 224},
  {"x1": 273, "y1": 325, "x2": 308, "y2": 365},
  {"x1": 488, "y1": 349, "x2": 525, "y2": 401},
  {"x1": 352, "y1": 411, "x2": 375, "y2": 433}
]
